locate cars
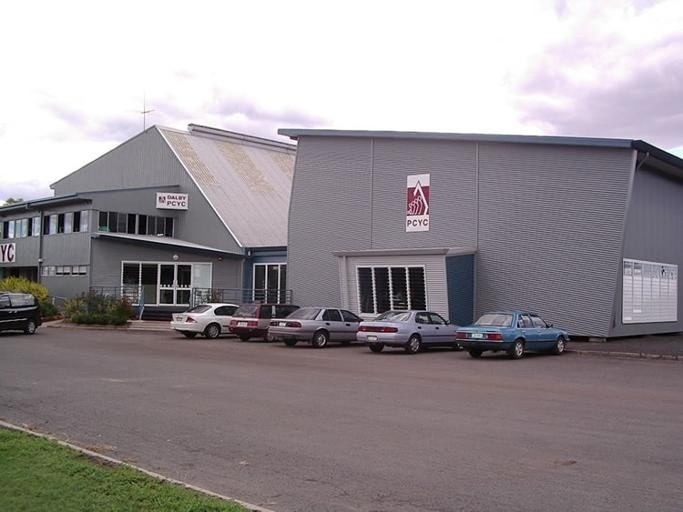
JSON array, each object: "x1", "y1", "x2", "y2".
[
  {"x1": 0, "y1": 292, "x2": 42, "y2": 335},
  {"x1": 169, "y1": 303, "x2": 464, "y2": 354},
  {"x1": 455, "y1": 311, "x2": 570, "y2": 359}
]
